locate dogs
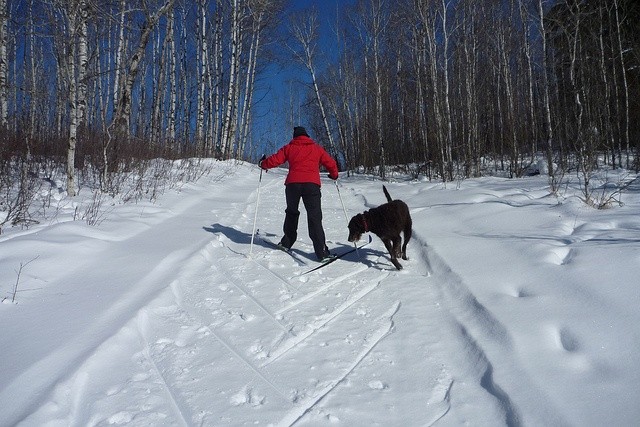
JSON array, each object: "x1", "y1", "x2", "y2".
[{"x1": 347, "y1": 185, "x2": 415, "y2": 272}]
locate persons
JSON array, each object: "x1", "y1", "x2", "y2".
[{"x1": 258, "y1": 125, "x2": 339, "y2": 261}]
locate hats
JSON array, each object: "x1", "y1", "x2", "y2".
[{"x1": 293, "y1": 126, "x2": 310, "y2": 137}]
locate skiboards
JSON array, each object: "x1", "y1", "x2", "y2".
[{"x1": 256, "y1": 228, "x2": 372, "y2": 275}]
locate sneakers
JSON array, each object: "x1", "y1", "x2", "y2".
[
  {"x1": 320, "y1": 252, "x2": 338, "y2": 263},
  {"x1": 278, "y1": 242, "x2": 289, "y2": 252}
]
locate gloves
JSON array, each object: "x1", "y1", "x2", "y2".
[
  {"x1": 327, "y1": 174, "x2": 339, "y2": 180},
  {"x1": 258, "y1": 154, "x2": 266, "y2": 169}
]
